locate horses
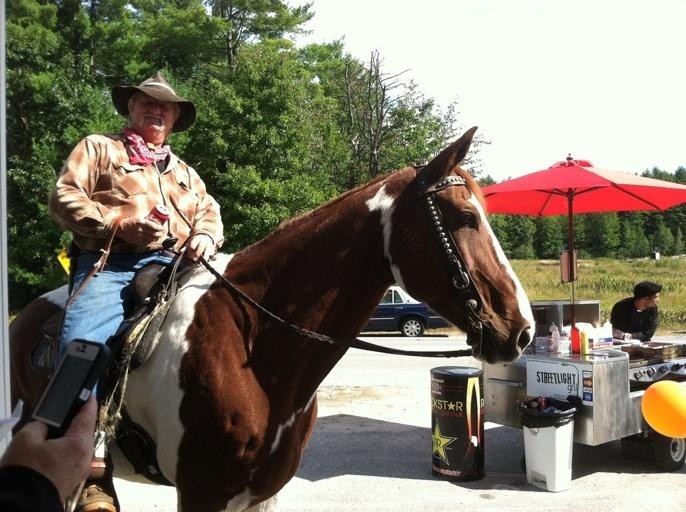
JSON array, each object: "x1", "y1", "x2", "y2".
[{"x1": 10, "y1": 125, "x2": 536, "y2": 512}]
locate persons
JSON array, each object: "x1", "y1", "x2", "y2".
[
  {"x1": 609, "y1": 280, "x2": 662, "y2": 343},
  {"x1": 48, "y1": 70, "x2": 226, "y2": 512},
  {"x1": 1, "y1": 340, "x2": 111, "y2": 512}
]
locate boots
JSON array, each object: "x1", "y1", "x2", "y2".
[{"x1": 75, "y1": 457, "x2": 120, "y2": 512}]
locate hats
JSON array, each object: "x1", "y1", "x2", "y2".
[
  {"x1": 111, "y1": 70, "x2": 197, "y2": 133},
  {"x1": 634, "y1": 282, "x2": 662, "y2": 299}
]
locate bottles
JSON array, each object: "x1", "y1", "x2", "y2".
[
  {"x1": 572, "y1": 327, "x2": 580, "y2": 353},
  {"x1": 603, "y1": 322, "x2": 613, "y2": 346},
  {"x1": 581, "y1": 330, "x2": 590, "y2": 357},
  {"x1": 142, "y1": 206, "x2": 168, "y2": 233},
  {"x1": 551, "y1": 324, "x2": 560, "y2": 353}
]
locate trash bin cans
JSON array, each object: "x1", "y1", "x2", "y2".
[{"x1": 520, "y1": 395, "x2": 583, "y2": 492}]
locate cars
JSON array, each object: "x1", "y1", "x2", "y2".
[{"x1": 355, "y1": 282, "x2": 457, "y2": 338}]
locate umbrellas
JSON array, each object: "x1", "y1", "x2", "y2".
[{"x1": 467, "y1": 156, "x2": 686, "y2": 324}]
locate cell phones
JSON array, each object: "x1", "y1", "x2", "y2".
[{"x1": 30, "y1": 338, "x2": 109, "y2": 438}]
